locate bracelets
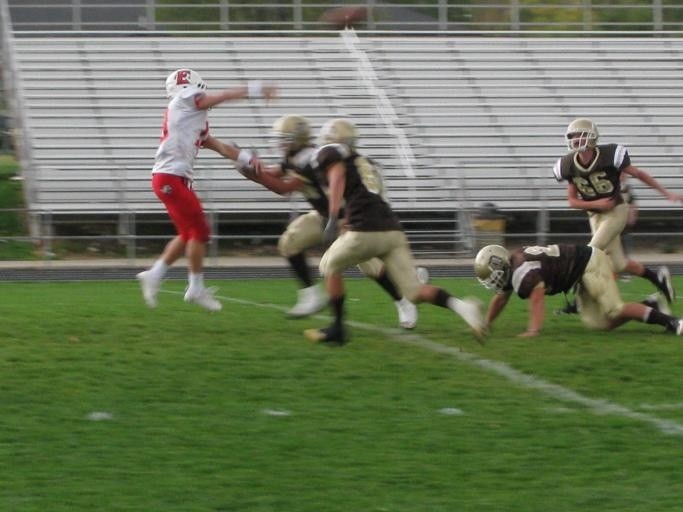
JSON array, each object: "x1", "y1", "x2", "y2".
[
  {"x1": 248, "y1": 82, "x2": 262, "y2": 97},
  {"x1": 237, "y1": 150, "x2": 252, "y2": 165}
]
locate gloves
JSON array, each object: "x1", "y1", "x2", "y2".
[{"x1": 322, "y1": 216, "x2": 336, "y2": 245}]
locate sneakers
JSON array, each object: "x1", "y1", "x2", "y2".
[
  {"x1": 184, "y1": 289, "x2": 222, "y2": 310},
  {"x1": 649, "y1": 265, "x2": 683, "y2": 335},
  {"x1": 287, "y1": 285, "x2": 324, "y2": 318},
  {"x1": 395, "y1": 296, "x2": 418, "y2": 330},
  {"x1": 306, "y1": 326, "x2": 347, "y2": 344},
  {"x1": 137, "y1": 271, "x2": 160, "y2": 309},
  {"x1": 461, "y1": 296, "x2": 490, "y2": 346}
]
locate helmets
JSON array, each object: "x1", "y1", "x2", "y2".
[
  {"x1": 165, "y1": 68, "x2": 207, "y2": 97},
  {"x1": 472, "y1": 245, "x2": 515, "y2": 298},
  {"x1": 320, "y1": 118, "x2": 356, "y2": 147},
  {"x1": 269, "y1": 117, "x2": 310, "y2": 152},
  {"x1": 566, "y1": 119, "x2": 600, "y2": 155}
]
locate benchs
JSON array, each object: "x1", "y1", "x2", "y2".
[{"x1": 1, "y1": 29, "x2": 682, "y2": 214}]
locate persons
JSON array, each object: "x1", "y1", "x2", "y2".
[
  {"x1": 612, "y1": 170, "x2": 638, "y2": 282},
  {"x1": 475, "y1": 243, "x2": 683, "y2": 336},
  {"x1": 135, "y1": 68, "x2": 279, "y2": 312},
  {"x1": 304, "y1": 118, "x2": 490, "y2": 347},
  {"x1": 239, "y1": 115, "x2": 418, "y2": 330},
  {"x1": 552, "y1": 119, "x2": 683, "y2": 316}
]
